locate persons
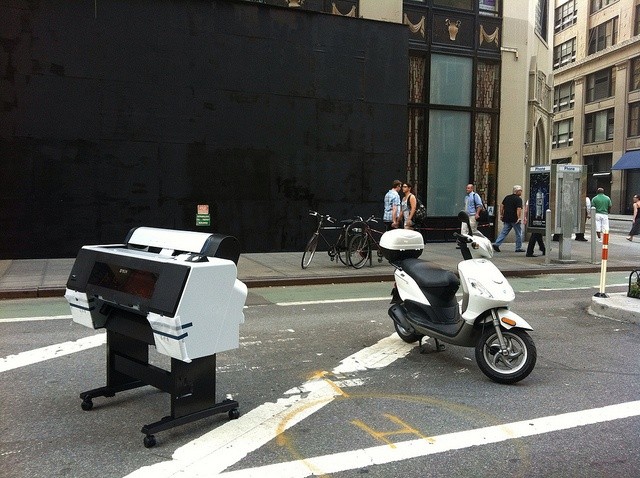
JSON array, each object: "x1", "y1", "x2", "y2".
[
  {"x1": 394, "y1": 182, "x2": 417, "y2": 230},
  {"x1": 382, "y1": 179, "x2": 403, "y2": 231},
  {"x1": 591, "y1": 187, "x2": 613, "y2": 243},
  {"x1": 492, "y1": 185, "x2": 526, "y2": 252},
  {"x1": 456, "y1": 182, "x2": 483, "y2": 249},
  {"x1": 534, "y1": 187, "x2": 545, "y2": 220},
  {"x1": 522, "y1": 199, "x2": 552, "y2": 257},
  {"x1": 575, "y1": 196, "x2": 591, "y2": 241},
  {"x1": 625, "y1": 193, "x2": 640, "y2": 243}
]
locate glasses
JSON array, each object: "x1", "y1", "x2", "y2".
[
  {"x1": 539, "y1": 191, "x2": 541, "y2": 193},
  {"x1": 403, "y1": 186, "x2": 409, "y2": 189},
  {"x1": 519, "y1": 191, "x2": 523, "y2": 193}
]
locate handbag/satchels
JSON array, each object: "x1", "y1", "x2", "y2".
[
  {"x1": 474, "y1": 192, "x2": 490, "y2": 226},
  {"x1": 408, "y1": 195, "x2": 428, "y2": 224}
]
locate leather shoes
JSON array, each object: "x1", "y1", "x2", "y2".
[
  {"x1": 526, "y1": 253, "x2": 539, "y2": 258},
  {"x1": 579, "y1": 238, "x2": 589, "y2": 242}
]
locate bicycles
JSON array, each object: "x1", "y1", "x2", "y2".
[
  {"x1": 301, "y1": 210, "x2": 365, "y2": 268},
  {"x1": 349, "y1": 215, "x2": 393, "y2": 268}
]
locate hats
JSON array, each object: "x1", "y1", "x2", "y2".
[{"x1": 514, "y1": 186, "x2": 523, "y2": 195}]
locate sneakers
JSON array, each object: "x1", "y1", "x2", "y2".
[
  {"x1": 597, "y1": 238, "x2": 603, "y2": 243},
  {"x1": 493, "y1": 244, "x2": 502, "y2": 253},
  {"x1": 516, "y1": 249, "x2": 527, "y2": 253}
]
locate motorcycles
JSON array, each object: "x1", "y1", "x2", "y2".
[{"x1": 379, "y1": 212, "x2": 536, "y2": 384}]
